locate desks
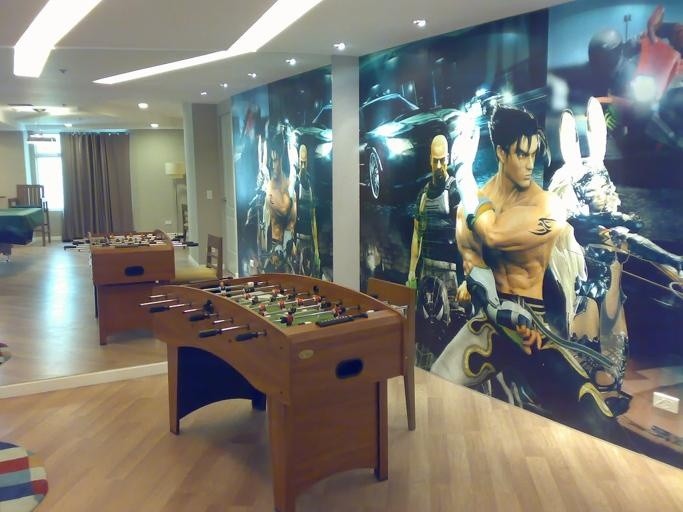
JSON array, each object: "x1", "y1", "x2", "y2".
[{"x1": 0, "y1": 207, "x2": 41, "y2": 244}]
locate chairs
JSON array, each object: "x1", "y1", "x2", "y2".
[
  {"x1": 363, "y1": 273, "x2": 418, "y2": 432},
  {"x1": 177, "y1": 234, "x2": 224, "y2": 284},
  {"x1": 8, "y1": 184, "x2": 52, "y2": 246}
]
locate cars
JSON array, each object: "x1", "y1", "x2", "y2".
[
  {"x1": 290, "y1": 104, "x2": 332, "y2": 163},
  {"x1": 360, "y1": 89, "x2": 514, "y2": 203}
]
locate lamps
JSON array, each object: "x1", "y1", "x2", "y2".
[
  {"x1": 165, "y1": 162, "x2": 182, "y2": 234},
  {"x1": 25, "y1": 107, "x2": 56, "y2": 142}
]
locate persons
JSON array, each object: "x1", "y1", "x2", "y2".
[
  {"x1": 234, "y1": 105, "x2": 320, "y2": 276},
  {"x1": 408, "y1": 6, "x2": 683, "y2": 440}
]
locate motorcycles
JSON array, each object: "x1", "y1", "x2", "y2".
[{"x1": 604, "y1": 5, "x2": 683, "y2": 159}]
